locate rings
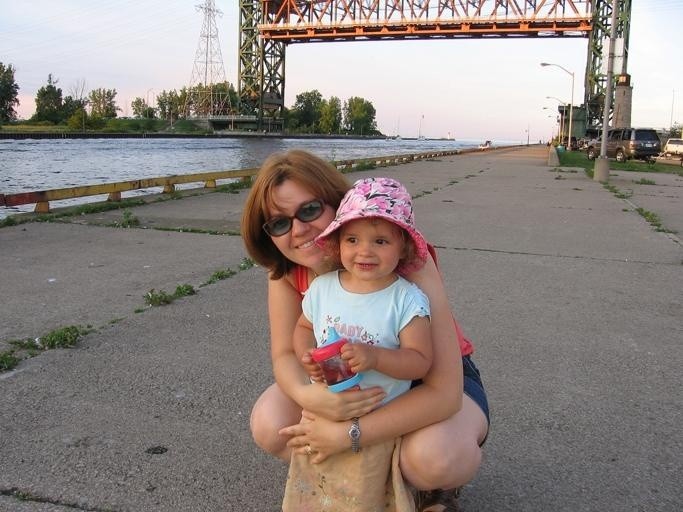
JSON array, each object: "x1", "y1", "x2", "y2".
[{"x1": 306, "y1": 444, "x2": 314, "y2": 453}]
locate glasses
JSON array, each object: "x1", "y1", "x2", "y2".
[{"x1": 262, "y1": 197, "x2": 326, "y2": 237}]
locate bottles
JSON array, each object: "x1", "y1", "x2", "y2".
[{"x1": 311, "y1": 326, "x2": 362, "y2": 394}]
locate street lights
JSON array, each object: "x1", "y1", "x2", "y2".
[
  {"x1": 540, "y1": 62, "x2": 575, "y2": 151},
  {"x1": 542, "y1": 94, "x2": 566, "y2": 147},
  {"x1": 419, "y1": 113, "x2": 425, "y2": 138}
]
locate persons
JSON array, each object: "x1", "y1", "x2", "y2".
[
  {"x1": 239, "y1": 148, "x2": 490, "y2": 512},
  {"x1": 278, "y1": 177, "x2": 435, "y2": 512}
]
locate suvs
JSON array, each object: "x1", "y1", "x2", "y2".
[
  {"x1": 664, "y1": 138, "x2": 682, "y2": 158},
  {"x1": 587, "y1": 126, "x2": 662, "y2": 165},
  {"x1": 555, "y1": 135, "x2": 579, "y2": 152}
]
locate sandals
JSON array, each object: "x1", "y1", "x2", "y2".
[{"x1": 414, "y1": 487, "x2": 461, "y2": 512}]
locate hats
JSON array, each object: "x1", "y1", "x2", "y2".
[{"x1": 314, "y1": 177, "x2": 428, "y2": 276}]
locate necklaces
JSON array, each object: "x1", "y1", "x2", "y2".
[{"x1": 311, "y1": 268, "x2": 318, "y2": 280}]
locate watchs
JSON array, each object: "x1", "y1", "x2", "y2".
[{"x1": 349, "y1": 418, "x2": 363, "y2": 451}]
